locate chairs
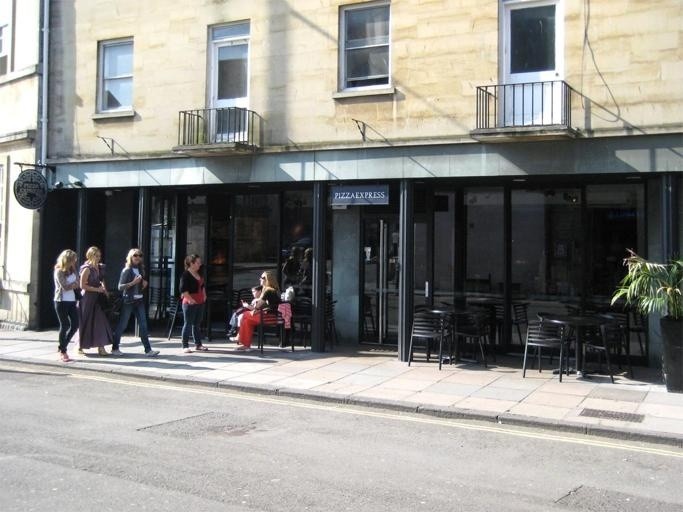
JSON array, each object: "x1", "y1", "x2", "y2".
[
  {"x1": 163, "y1": 284, "x2": 339, "y2": 354},
  {"x1": 407, "y1": 301, "x2": 650, "y2": 384}
]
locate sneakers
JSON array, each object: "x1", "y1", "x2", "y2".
[
  {"x1": 98, "y1": 348, "x2": 110, "y2": 357},
  {"x1": 111, "y1": 350, "x2": 126, "y2": 357},
  {"x1": 236, "y1": 345, "x2": 248, "y2": 350},
  {"x1": 229, "y1": 337, "x2": 238, "y2": 341},
  {"x1": 144, "y1": 350, "x2": 161, "y2": 357},
  {"x1": 226, "y1": 330, "x2": 236, "y2": 337},
  {"x1": 78, "y1": 349, "x2": 87, "y2": 356}
]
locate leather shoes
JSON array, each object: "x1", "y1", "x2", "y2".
[
  {"x1": 195, "y1": 346, "x2": 208, "y2": 351},
  {"x1": 183, "y1": 347, "x2": 192, "y2": 353}
]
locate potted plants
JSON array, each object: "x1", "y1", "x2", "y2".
[{"x1": 611, "y1": 249, "x2": 683, "y2": 393}]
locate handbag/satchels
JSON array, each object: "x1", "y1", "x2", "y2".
[
  {"x1": 111, "y1": 295, "x2": 124, "y2": 315},
  {"x1": 73, "y1": 287, "x2": 83, "y2": 300}
]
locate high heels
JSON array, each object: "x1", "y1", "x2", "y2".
[{"x1": 60, "y1": 351, "x2": 70, "y2": 362}]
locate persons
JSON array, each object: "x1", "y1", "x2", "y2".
[
  {"x1": 282, "y1": 246, "x2": 304, "y2": 293},
  {"x1": 75, "y1": 245, "x2": 113, "y2": 356},
  {"x1": 177, "y1": 254, "x2": 207, "y2": 352},
  {"x1": 111, "y1": 248, "x2": 160, "y2": 357},
  {"x1": 295, "y1": 248, "x2": 313, "y2": 297},
  {"x1": 227, "y1": 272, "x2": 281, "y2": 350},
  {"x1": 54, "y1": 249, "x2": 79, "y2": 361}
]
